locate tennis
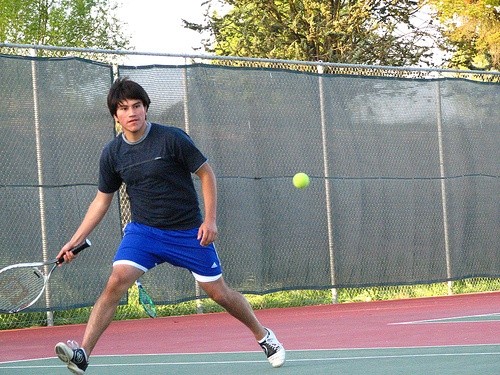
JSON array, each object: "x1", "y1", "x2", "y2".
[{"x1": 291, "y1": 173, "x2": 311, "y2": 190}]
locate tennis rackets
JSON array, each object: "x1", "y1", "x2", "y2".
[
  {"x1": 0, "y1": 238, "x2": 91, "y2": 314},
  {"x1": 137, "y1": 278, "x2": 156, "y2": 318}
]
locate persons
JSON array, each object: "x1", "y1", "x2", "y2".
[{"x1": 53, "y1": 76, "x2": 287, "y2": 375}]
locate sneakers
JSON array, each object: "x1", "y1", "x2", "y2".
[
  {"x1": 53, "y1": 340, "x2": 90, "y2": 375},
  {"x1": 255, "y1": 326, "x2": 285, "y2": 367}
]
